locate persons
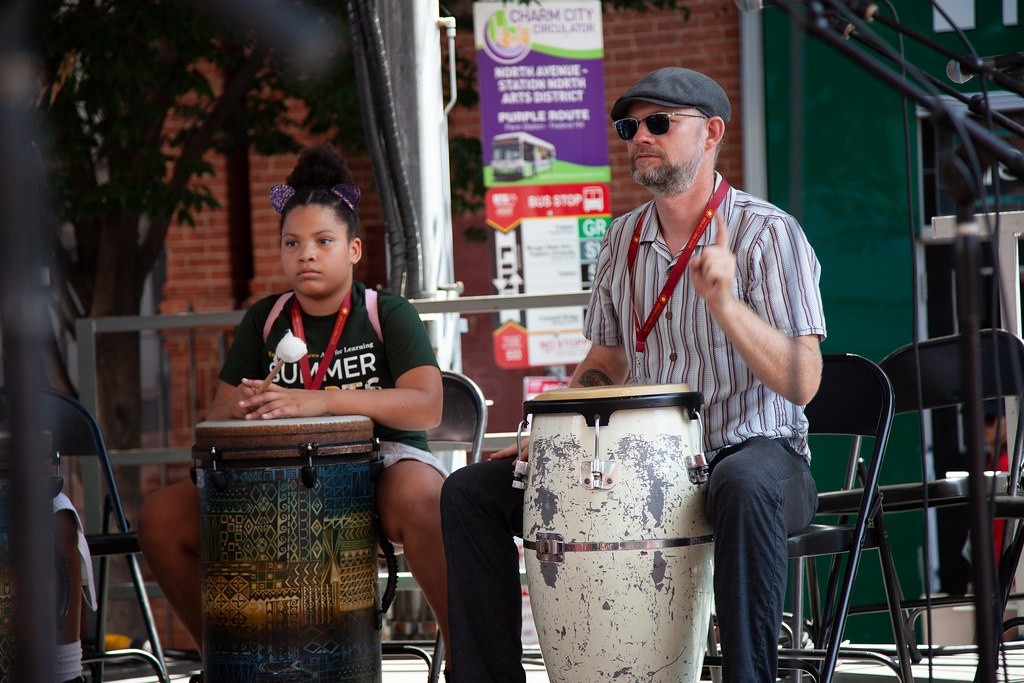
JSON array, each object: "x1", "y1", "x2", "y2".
[
  {"x1": 53, "y1": 492, "x2": 98, "y2": 683},
  {"x1": 438, "y1": 66, "x2": 827, "y2": 683},
  {"x1": 138, "y1": 145, "x2": 452, "y2": 683},
  {"x1": 962, "y1": 399, "x2": 1015, "y2": 593}
]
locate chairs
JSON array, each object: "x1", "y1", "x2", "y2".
[
  {"x1": 41, "y1": 385, "x2": 170, "y2": 683},
  {"x1": 710, "y1": 327, "x2": 1024, "y2": 683},
  {"x1": 380, "y1": 370, "x2": 488, "y2": 683}
]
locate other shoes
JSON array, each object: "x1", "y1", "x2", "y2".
[{"x1": 189, "y1": 670, "x2": 202, "y2": 683}]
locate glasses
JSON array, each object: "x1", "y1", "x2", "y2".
[{"x1": 612, "y1": 112, "x2": 708, "y2": 142}]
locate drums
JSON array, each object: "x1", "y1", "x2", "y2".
[
  {"x1": 191, "y1": 413, "x2": 386, "y2": 682},
  {"x1": 519, "y1": 381, "x2": 718, "y2": 682}
]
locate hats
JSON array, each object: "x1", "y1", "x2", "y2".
[{"x1": 611, "y1": 67, "x2": 732, "y2": 125}]
